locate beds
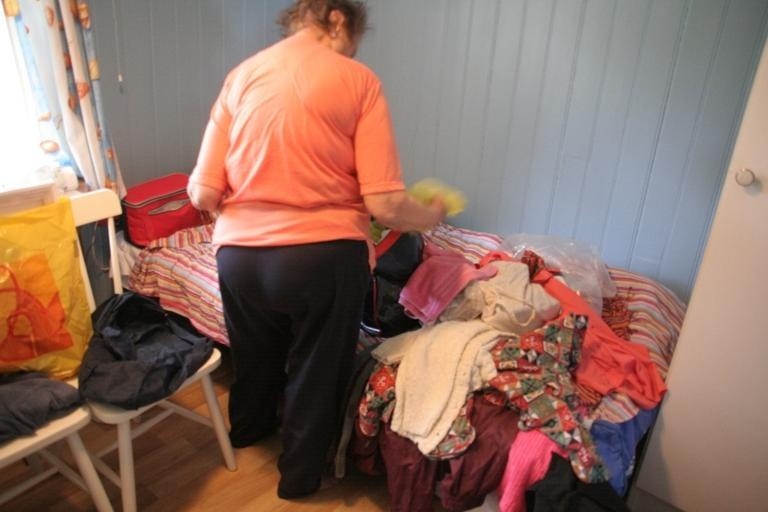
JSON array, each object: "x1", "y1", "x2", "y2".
[{"x1": 106, "y1": 160, "x2": 691, "y2": 512}]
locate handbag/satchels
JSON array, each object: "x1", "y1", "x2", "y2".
[
  {"x1": 476, "y1": 260, "x2": 561, "y2": 334},
  {"x1": 77, "y1": 288, "x2": 213, "y2": 410},
  {"x1": 121, "y1": 173, "x2": 212, "y2": 247}
]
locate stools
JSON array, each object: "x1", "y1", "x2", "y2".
[{"x1": 1, "y1": 344, "x2": 114, "y2": 512}]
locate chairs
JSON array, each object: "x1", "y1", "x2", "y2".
[{"x1": 55, "y1": 187, "x2": 238, "y2": 512}]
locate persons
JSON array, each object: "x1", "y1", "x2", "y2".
[{"x1": 185, "y1": 2, "x2": 447, "y2": 500}]
